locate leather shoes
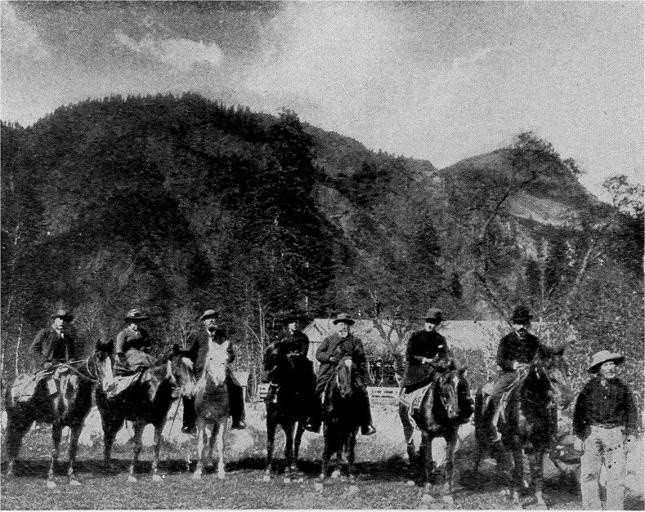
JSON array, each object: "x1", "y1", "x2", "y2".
[
  {"x1": 181, "y1": 426, "x2": 195, "y2": 433},
  {"x1": 233, "y1": 421, "x2": 245, "y2": 429}
]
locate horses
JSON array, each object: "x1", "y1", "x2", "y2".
[
  {"x1": 192, "y1": 336, "x2": 231, "y2": 479},
  {"x1": 319, "y1": 353, "x2": 363, "y2": 484},
  {"x1": 3, "y1": 339, "x2": 117, "y2": 489},
  {"x1": 473, "y1": 343, "x2": 572, "y2": 507},
  {"x1": 262, "y1": 340, "x2": 316, "y2": 484},
  {"x1": 398, "y1": 360, "x2": 469, "y2": 503},
  {"x1": 95, "y1": 338, "x2": 197, "y2": 485}
]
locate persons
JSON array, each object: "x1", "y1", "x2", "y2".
[
  {"x1": 399, "y1": 309, "x2": 450, "y2": 445},
  {"x1": 27, "y1": 307, "x2": 75, "y2": 431},
  {"x1": 306, "y1": 312, "x2": 376, "y2": 435},
  {"x1": 181, "y1": 310, "x2": 247, "y2": 433},
  {"x1": 481, "y1": 306, "x2": 559, "y2": 439},
  {"x1": 115, "y1": 308, "x2": 156, "y2": 403},
  {"x1": 263, "y1": 312, "x2": 318, "y2": 404},
  {"x1": 573, "y1": 349, "x2": 638, "y2": 511}
]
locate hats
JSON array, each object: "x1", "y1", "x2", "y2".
[
  {"x1": 51, "y1": 309, "x2": 73, "y2": 321},
  {"x1": 124, "y1": 309, "x2": 149, "y2": 322},
  {"x1": 333, "y1": 312, "x2": 354, "y2": 324},
  {"x1": 587, "y1": 350, "x2": 625, "y2": 372},
  {"x1": 200, "y1": 309, "x2": 220, "y2": 320},
  {"x1": 421, "y1": 307, "x2": 445, "y2": 320},
  {"x1": 282, "y1": 310, "x2": 304, "y2": 322},
  {"x1": 506, "y1": 306, "x2": 534, "y2": 322}
]
